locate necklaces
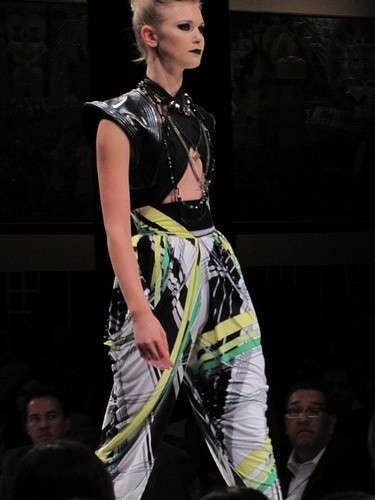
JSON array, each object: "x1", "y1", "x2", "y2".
[
  {"x1": 137, "y1": 82, "x2": 214, "y2": 210},
  {"x1": 137, "y1": 80, "x2": 211, "y2": 195},
  {"x1": 138, "y1": 79, "x2": 204, "y2": 160}
]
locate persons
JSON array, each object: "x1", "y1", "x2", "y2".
[
  {"x1": 7, "y1": 385, "x2": 87, "y2": 462},
  {"x1": 0, "y1": 437, "x2": 117, "y2": 500},
  {"x1": 84, "y1": 0, "x2": 286, "y2": 500},
  {"x1": 197, "y1": 486, "x2": 269, "y2": 500},
  {"x1": 268, "y1": 376, "x2": 375, "y2": 500}
]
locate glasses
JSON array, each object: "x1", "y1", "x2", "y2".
[{"x1": 283, "y1": 405, "x2": 324, "y2": 419}]
